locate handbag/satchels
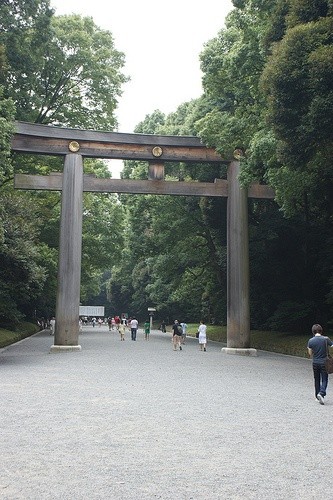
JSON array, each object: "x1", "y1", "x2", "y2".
[
  {"x1": 196, "y1": 332, "x2": 199, "y2": 338},
  {"x1": 325, "y1": 358, "x2": 333, "y2": 374},
  {"x1": 177, "y1": 326, "x2": 183, "y2": 334}
]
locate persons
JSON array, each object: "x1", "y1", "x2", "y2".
[
  {"x1": 122, "y1": 317, "x2": 131, "y2": 326},
  {"x1": 307, "y1": 324, "x2": 333, "y2": 404},
  {"x1": 180, "y1": 320, "x2": 188, "y2": 345},
  {"x1": 144, "y1": 321, "x2": 150, "y2": 341},
  {"x1": 84, "y1": 317, "x2": 87, "y2": 325},
  {"x1": 108, "y1": 316, "x2": 120, "y2": 331},
  {"x1": 119, "y1": 320, "x2": 125, "y2": 341},
  {"x1": 131, "y1": 316, "x2": 138, "y2": 341},
  {"x1": 197, "y1": 320, "x2": 207, "y2": 351},
  {"x1": 50, "y1": 317, "x2": 55, "y2": 336},
  {"x1": 79, "y1": 318, "x2": 83, "y2": 333},
  {"x1": 92, "y1": 317, "x2": 96, "y2": 327},
  {"x1": 161, "y1": 321, "x2": 166, "y2": 332},
  {"x1": 172, "y1": 319, "x2": 182, "y2": 351},
  {"x1": 98, "y1": 318, "x2": 102, "y2": 328}
]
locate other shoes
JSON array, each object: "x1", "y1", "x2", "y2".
[
  {"x1": 179, "y1": 347, "x2": 182, "y2": 350},
  {"x1": 200, "y1": 348, "x2": 202, "y2": 351},
  {"x1": 204, "y1": 347, "x2": 206, "y2": 351},
  {"x1": 316, "y1": 399, "x2": 319, "y2": 402},
  {"x1": 317, "y1": 394, "x2": 324, "y2": 405}
]
